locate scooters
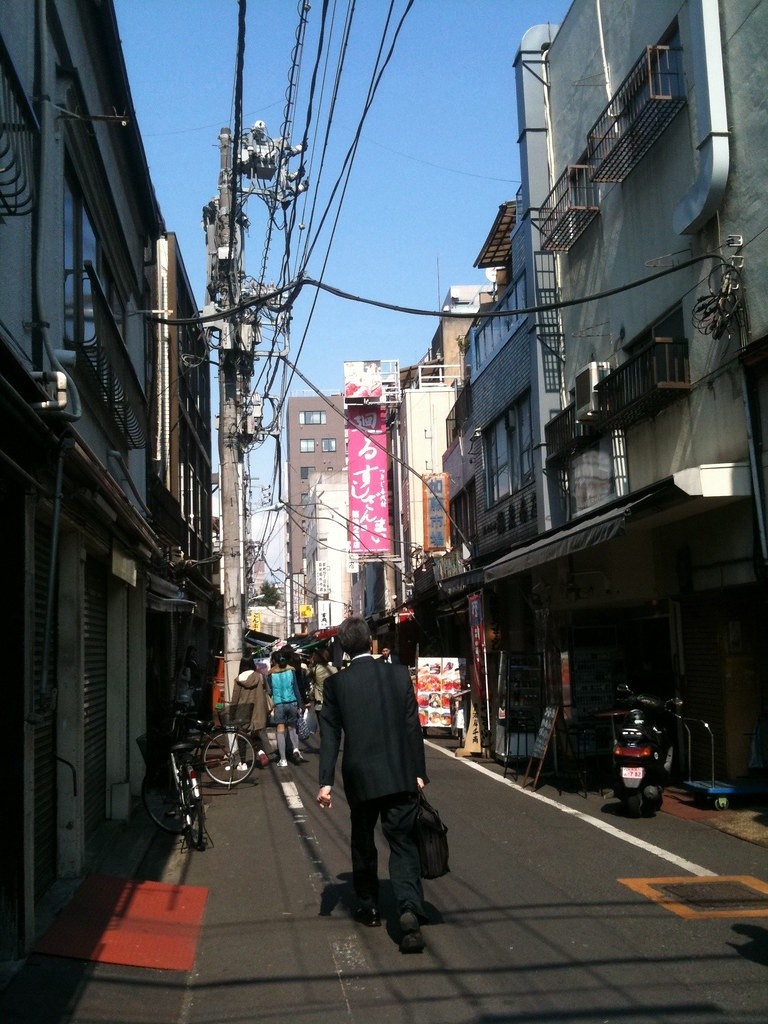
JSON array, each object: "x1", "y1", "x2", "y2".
[{"x1": 610, "y1": 684, "x2": 684, "y2": 818}]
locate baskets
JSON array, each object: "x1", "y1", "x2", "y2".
[
  {"x1": 217, "y1": 703, "x2": 254, "y2": 728},
  {"x1": 136, "y1": 730, "x2": 163, "y2": 768}
]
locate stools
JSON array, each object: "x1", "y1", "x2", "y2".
[
  {"x1": 557, "y1": 729, "x2": 601, "y2": 798},
  {"x1": 504, "y1": 714, "x2": 539, "y2": 781},
  {"x1": 593, "y1": 708, "x2": 628, "y2": 767}
]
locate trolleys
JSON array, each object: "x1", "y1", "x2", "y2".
[{"x1": 679, "y1": 717, "x2": 768, "y2": 812}]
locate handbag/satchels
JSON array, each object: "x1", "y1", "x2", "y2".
[
  {"x1": 189, "y1": 670, "x2": 211, "y2": 704},
  {"x1": 264, "y1": 689, "x2": 275, "y2": 714},
  {"x1": 298, "y1": 706, "x2": 317, "y2": 741},
  {"x1": 415, "y1": 787, "x2": 451, "y2": 880}
]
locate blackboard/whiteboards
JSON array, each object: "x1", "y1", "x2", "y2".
[{"x1": 531, "y1": 705, "x2": 575, "y2": 759}]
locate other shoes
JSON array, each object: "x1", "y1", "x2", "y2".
[
  {"x1": 292, "y1": 748, "x2": 304, "y2": 764},
  {"x1": 236, "y1": 762, "x2": 248, "y2": 771},
  {"x1": 276, "y1": 759, "x2": 288, "y2": 767},
  {"x1": 258, "y1": 750, "x2": 269, "y2": 766}
]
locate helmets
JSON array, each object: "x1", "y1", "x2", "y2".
[{"x1": 622, "y1": 708, "x2": 648, "y2": 728}]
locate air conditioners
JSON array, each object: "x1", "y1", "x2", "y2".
[{"x1": 573, "y1": 360, "x2": 611, "y2": 420}]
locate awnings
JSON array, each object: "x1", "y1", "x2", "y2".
[{"x1": 482, "y1": 494, "x2": 655, "y2": 583}]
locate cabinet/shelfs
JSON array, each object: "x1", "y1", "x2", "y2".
[{"x1": 491, "y1": 651, "x2": 546, "y2": 766}]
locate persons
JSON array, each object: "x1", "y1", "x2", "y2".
[
  {"x1": 266, "y1": 651, "x2": 304, "y2": 767},
  {"x1": 316, "y1": 617, "x2": 430, "y2": 952},
  {"x1": 307, "y1": 649, "x2": 339, "y2": 722},
  {"x1": 277, "y1": 644, "x2": 311, "y2": 707},
  {"x1": 378, "y1": 643, "x2": 400, "y2": 664},
  {"x1": 229, "y1": 654, "x2": 269, "y2": 771},
  {"x1": 184, "y1": 646, "x2": 198, "y2": 675}
]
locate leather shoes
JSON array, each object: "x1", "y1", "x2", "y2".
[
  {"x1": 351, "y1": 901, "x2": 380, "y2": 926},
  {"x1": 400, "y1": 909, "x2": 424, "y2": 952}
]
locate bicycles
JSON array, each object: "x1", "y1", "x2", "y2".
[
  {"x1": 157, "y1": 697, "x2": 256, "y2": 801},
  {"x1": 135, "y1": 709, "x2": 215, "y2": 854}
]
